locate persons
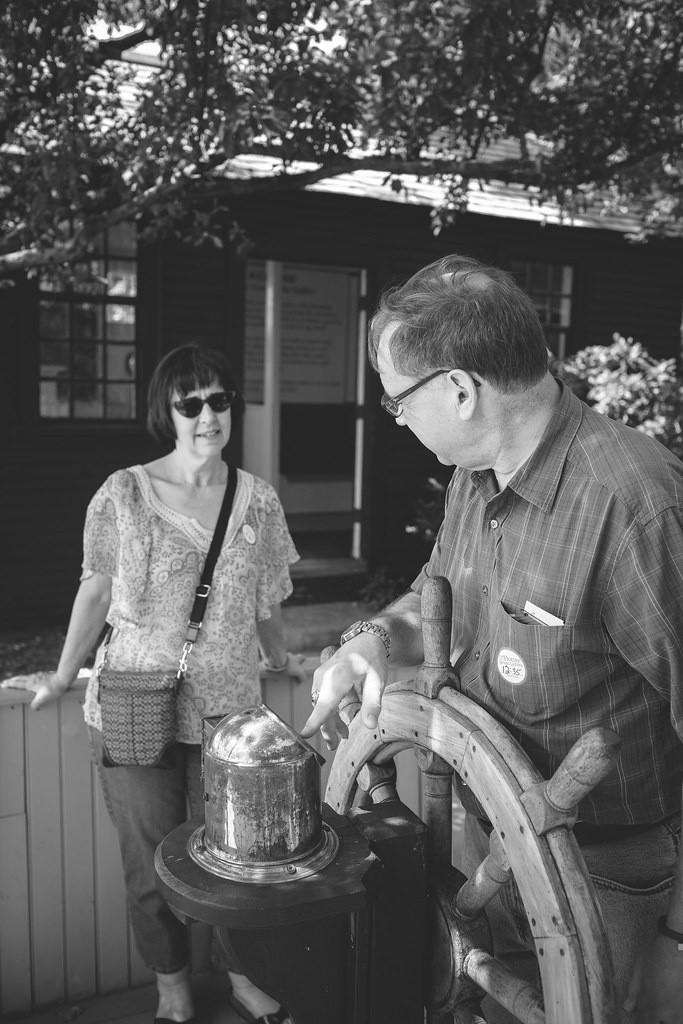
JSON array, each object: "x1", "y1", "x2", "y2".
[
  {"x1": 0, "y1": 345, "x2": 307, "y2": 1024},
  {"x1": 300, "y1": 254, "x2": 683, "y2": 1024}
]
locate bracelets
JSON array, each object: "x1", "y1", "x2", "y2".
[{"x1": 262, "y1": 654, "x2": 291, "y2": 672}]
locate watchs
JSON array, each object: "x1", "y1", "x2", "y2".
[{"x1": 340, "y1": 620, "x2": 392, "y2": 660}]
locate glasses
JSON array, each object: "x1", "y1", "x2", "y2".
[
  {"x1": 169, "y1": 389, "x2": 238, "y2": 418},
  {"x1": 381, "y1": 370, "x2": 481, "y2": 418}
]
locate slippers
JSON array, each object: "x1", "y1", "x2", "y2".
[
  {"x1": 229, "y1": 986, "x2": 294, "y2": 1024},
  {"x1": 154, "y1": 988, "x2": 197, "y2": 1024}
]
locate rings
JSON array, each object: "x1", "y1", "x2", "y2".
[{"x1": 311, "y1": 689, "x2": 320, "y2": 707}]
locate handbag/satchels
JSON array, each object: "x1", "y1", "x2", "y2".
[{"x1": 96, "y1": 667, "x2": 176, "y2": 768}]
